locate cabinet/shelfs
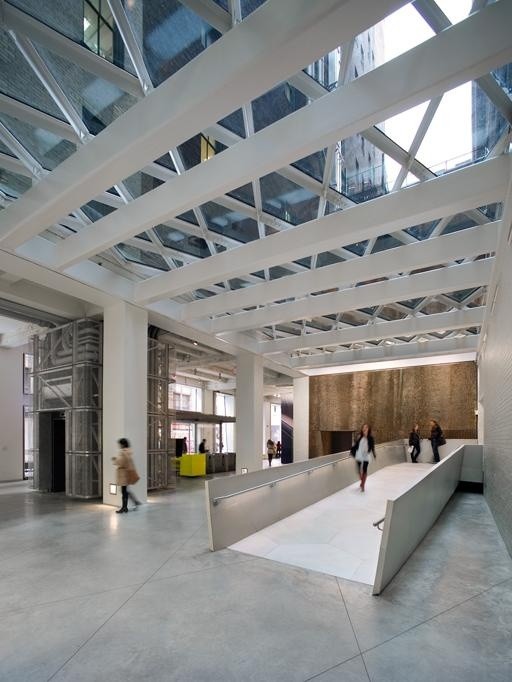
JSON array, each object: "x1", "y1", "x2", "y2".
[{"x1": 179, "y1": 453, "x2": 207, "y2": 477}]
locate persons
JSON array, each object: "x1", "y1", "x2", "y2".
[
  {"x1": 112, "y1": 437, "x2": 142, "y2": 514},
  {"x1": 408, "y1": 423, "x2": 423, "y2": 463},
  {"x1": 183, "y1": 437, "x2": 188, "y2": 453},
  {"x1": 426, "y1": 418, "x2": 444, "y2": 464},
  {"x1": 198, "y1": 438, "x2": 207, "y2": 453},
  {"x1": 266, "y1": 439, "x2": 275, "y2": 466},
  {"x1": 275, "y1": 441, "x2": 282, "y2": 458},
  {"x1": 349, "y1": 423, "x2": 377, "y2": 494}
]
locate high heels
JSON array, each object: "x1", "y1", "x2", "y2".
[
  {"x1": 359, "y1": 472, "x2": 366, "y2": 492},
  {"x1": 116, "y1": 507, "x2": 128, "y2": 513}
]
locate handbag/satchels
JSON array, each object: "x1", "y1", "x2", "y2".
[
  {"x1": 408, "y1": 445, "x2": 414, "y2": 453},
  {"x1": 128, "y1": 469, "x2": 140, "y2": 484},
  {"x1": 351, "y1": 445, "x2": 356, "y2": 457},
  {"x1": 438, "y1": 436, "x2": 446, "y2": 446}
]
